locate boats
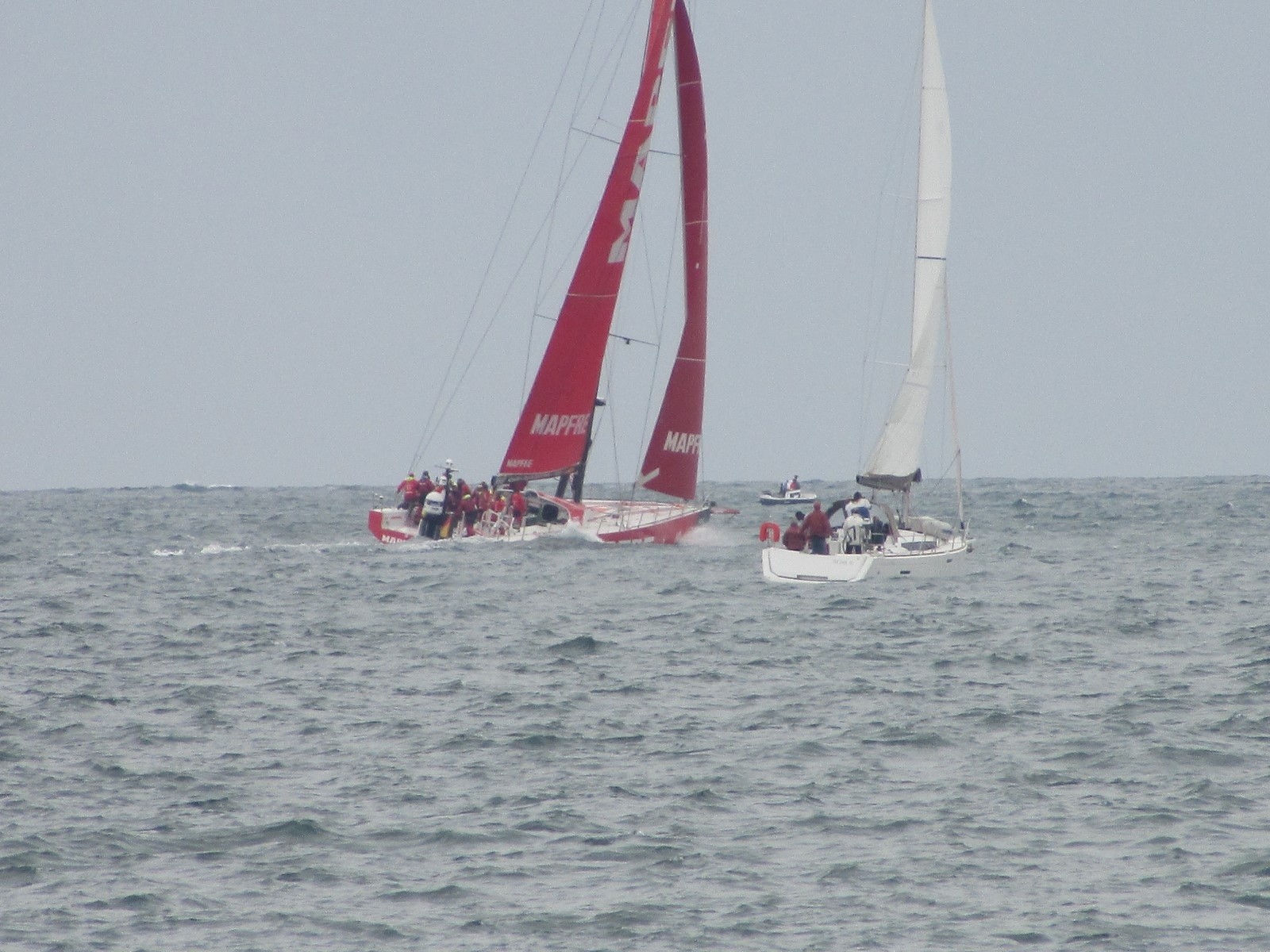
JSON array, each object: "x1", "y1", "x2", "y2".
[{"x1": 758, "y1": 481, "x2": 818, "y2": 505}]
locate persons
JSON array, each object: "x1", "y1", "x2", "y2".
[
  {"x1": 795, "y1": 511, "x2": 808, "y2": 544},
  {"x1": 801, "y1": 501, "x2": 833, "y2": 555},
  {"x1": 396, "y1": 471, "x2": 527, "y2": 539},
  {"x1": 779, "y1": 475, "x2": 801, "y2": 498},
  {"x1": 783, "y1": 521, "x2": 806, "y2": 551},
  {"x1": 843, "y1": 508, "x2": 866, "y2": 554},
  {"x1": 845, "y1": 492, "x2": 892, "y2": 544}
]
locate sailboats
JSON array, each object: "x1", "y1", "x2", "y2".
[
  {"x1": 756, "y1": 0, "x2": 980, "y2": 589},
  {"x1": 357, "y1": 1, "x2": 725, "y2": 549}
]
